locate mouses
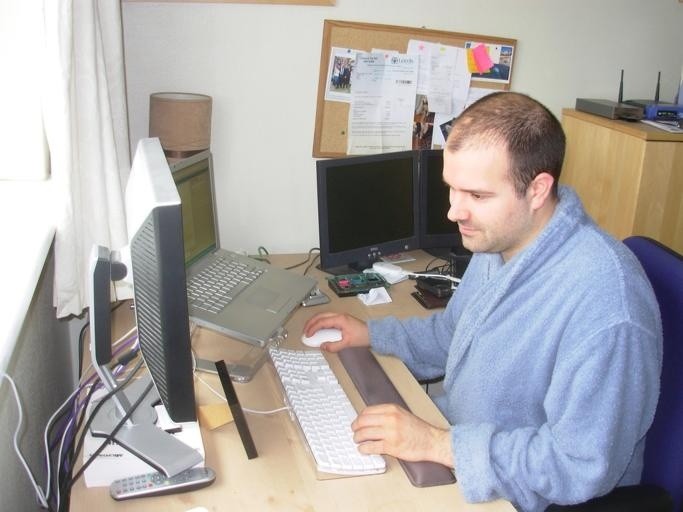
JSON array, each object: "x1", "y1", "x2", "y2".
[{"x1": 300, "y1": 326, "x2": 341, "y2": 349}]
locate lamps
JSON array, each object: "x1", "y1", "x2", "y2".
[{"x1": 149, "y1": 92, "x2": 212, "y2": 164}]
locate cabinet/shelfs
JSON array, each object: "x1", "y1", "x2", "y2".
[{"x1": 558, "y1": 108, "x2": 682, "y2": 256}]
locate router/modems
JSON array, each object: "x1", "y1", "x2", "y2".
[{"x1": 617, "y1": 67, "x2": 683, "y2": 122}]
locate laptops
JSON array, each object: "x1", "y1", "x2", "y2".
[{"x1": 169, "y1": 149, "x2": 321, "y2": 349}]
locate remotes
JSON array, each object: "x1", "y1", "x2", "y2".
[{"x1": 107, "y1": 465, "x2": 217, "y2": 500}]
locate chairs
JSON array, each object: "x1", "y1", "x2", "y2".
[{"x1": 544, "y1": 236, "x2": 683, "y2": 510}]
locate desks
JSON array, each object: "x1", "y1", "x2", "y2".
[{"x1": 69, "y1": 249, "x2": 514, "y2": 512}]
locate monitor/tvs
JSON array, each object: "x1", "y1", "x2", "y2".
[
  {"x1": 419, "y1": 149, "x2": 472, "y2": 260},
  {"x1": 0, "y1": 227, "x2": 81, "y2": 504},
  {"x1": 86, "y1": 136, "x2": 206, "y2": 480},
  {"x1": 316, "y1": 149, "x2": 421, "y2": 275}
]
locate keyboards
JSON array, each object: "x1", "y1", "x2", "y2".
[{"x1": 264, "y1": 342, "x2": 388, "y2": 481}]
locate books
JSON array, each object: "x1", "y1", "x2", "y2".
[{"x1": 379, "y1": 251, "x2": 416, "y2": 264}]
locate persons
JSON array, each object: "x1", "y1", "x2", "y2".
[
  {"x1": 332, "y1": 59, "x2": 351, "y2": 88},
  {"x1": 301, "y1": 92, "x2": 663, "y2": 512}
]
